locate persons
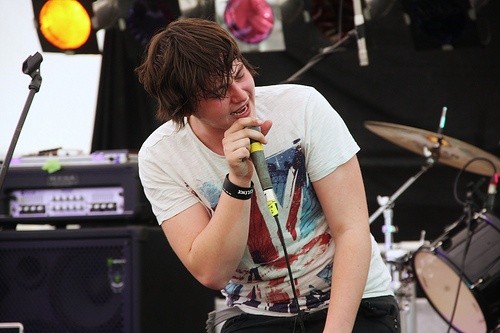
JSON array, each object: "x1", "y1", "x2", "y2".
[{"x1": 135, "y1": 19, "x2": 401, "y2": 333}]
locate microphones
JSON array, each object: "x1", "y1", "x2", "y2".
[
  {"x1": 353, "y1": 1, "x2": 369, "y2": 66},
  {"x1": 484, "y1": 172, "x2": 498, "y2": 213},
  {"x1": 243, "y1": 125, "x2": 280, "y2": 217}
]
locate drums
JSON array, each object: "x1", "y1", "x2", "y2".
[{"x1": 412, "y1": 210, "x2": 500, "y2": 333}]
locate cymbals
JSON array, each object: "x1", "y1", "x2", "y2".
[{"x1": 366, "y1": 120, "x2": 500, "y2": 177}]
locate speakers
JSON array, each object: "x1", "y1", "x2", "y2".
[{"x1": 0, "y1": 226, "x2": 214, "y2": 333}]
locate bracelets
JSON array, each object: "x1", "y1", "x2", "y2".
[{"x1": 222, "y1": 174, "x2": 254, "y2": 200}]
